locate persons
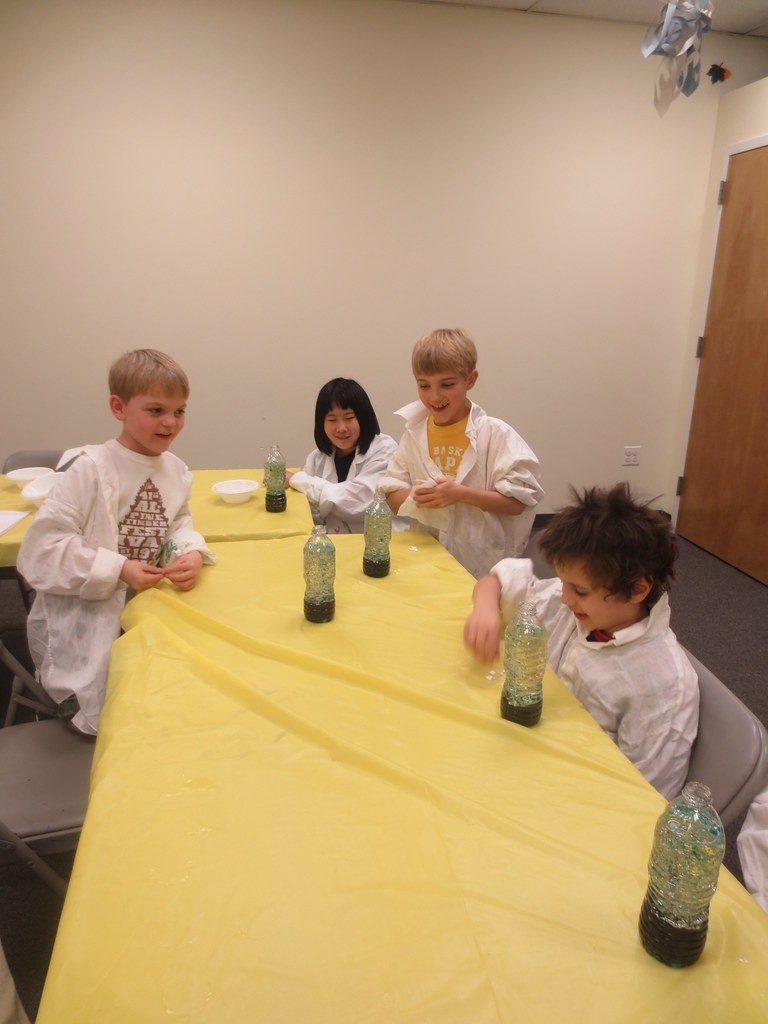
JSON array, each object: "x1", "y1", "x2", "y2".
[
  {"x1": 464, "y1": 481, "x2": 700, "y2": 801},
  {"x1": 383, "y1": 329, "x2": 546, "y2": 583},
  {"x1": 262, "y1": 378, "x2": 411, "y2": 535},
  {"x1": 17, "y1": 348, "x2": 216, "y2": 737}
]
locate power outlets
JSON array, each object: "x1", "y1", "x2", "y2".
[{"x1": 623, "y1": 446, "x2": 641, "y2": 466}]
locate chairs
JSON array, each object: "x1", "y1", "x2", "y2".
[
  {"x1": 0, "y1": 640, "x2": 96, "y2": 898},
  {"x1": 521, "y1": 529, "x2": 559, "y2": 579},
  {"x1": 679, "y1": 643, "x2": 768, "y2": 826}
]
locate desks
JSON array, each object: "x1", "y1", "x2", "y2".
[{"x1": 0, "y1": 468, "x2": 768, "y2": 1024}]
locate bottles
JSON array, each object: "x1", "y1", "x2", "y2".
[
  {"x1": 500, "y1": 601, "x2": 547, "y2": 726},
  {"x1": 639, "y1": 781, "x2": 725, "y2": 968},
  {"x1": 363, "y1": 492, "x2": 392, "y2": 578},
  {"x1": 264, "y1": 445, "x2": 287, "y2": 513},
  {"x1": 303, "y1": 525, "x2": 336, "y2": 623}
]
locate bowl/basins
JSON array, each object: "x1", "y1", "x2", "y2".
[
  {"x1": 212, "y1": 480, "x2": 259, "y2": 504},
  {"x1": 21, "y1": 472, "x2": 64, "y2": 506},
  {"x1": 6, "y1": 467, "x2": 53, "y2": 489}
]
